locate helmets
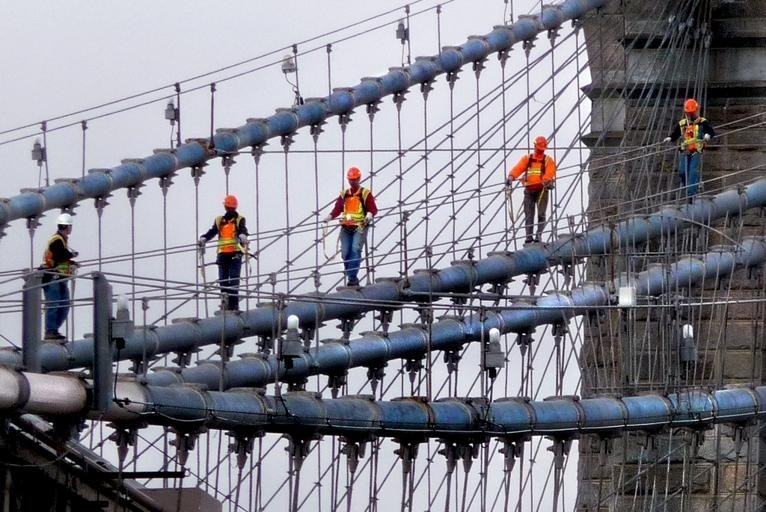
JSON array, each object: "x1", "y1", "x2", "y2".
[
  {"x1": 346, "y1": 167, "x2": 361, "y2": 179},
  {"x1": 534, "y1": 136, "x2": 547, "y2": 151},
  {"x1": 683, "y1": 98, "x2": 699, "y2": 113},
  {"x1": 223, "y1": 196, "x2": 239, "y2": 208},
  {"x1": 57, "y1": 213, "x2": 73, "y2": 225}
]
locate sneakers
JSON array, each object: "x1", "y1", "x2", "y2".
[
  {"x1": 525, "y1": 236, "x2": 539, "y2": 243},
  {"x1": 43, "y1": 336, "x2": 66, "y2": 343}
]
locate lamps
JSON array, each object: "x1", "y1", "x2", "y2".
[
  {"x1": 281, "y1": 315, "x2": 303, "y2": 359},
  {"x1": 484, "y1": 326, "x2": 506, "y2": 369},
  {"x1": 679, "y1": 323, "x2": 698, "y2": 363},
  {"x1": 108, "y1": 294, "x2": 137, "y2": 344}
]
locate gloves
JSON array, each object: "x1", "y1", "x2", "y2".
[
  {"x1": 664, "y1": 137, "x2": 672, "y2": 143},
  {"x1": 507, "y1": 176, "x2": 513, "y2": 186},
  {"x1": 200, "y1": 237, "x2": 207, "y2": 245},
  {"x1": 239, "y1": 234, "x2": 248, "y2": 244},
  {"x1": 365, "y1": 212, "x2": 372, "y2": 223},
  {"x1": 323, "y1": 216, "x2": 332, "y2": 221},
  {"x1": 704, "y1": 133, "x2": 711, "y2": 141}
]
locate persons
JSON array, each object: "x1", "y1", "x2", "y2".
[
  {"x1": 38, "y1": 213, "x2": 80, "y2": 339},
  {"x1": 505, "y1": 136, "x2": 557, "y2": 243},
  {"x1": 662, "y1": 99, "x2": 715, "y2": 205},
  {"x1": 323, "y1": 167, "x2": 378, "y2": 286},
  {"x1": 197, "y1": 195, "x2": 248, "y2": 309}
]
